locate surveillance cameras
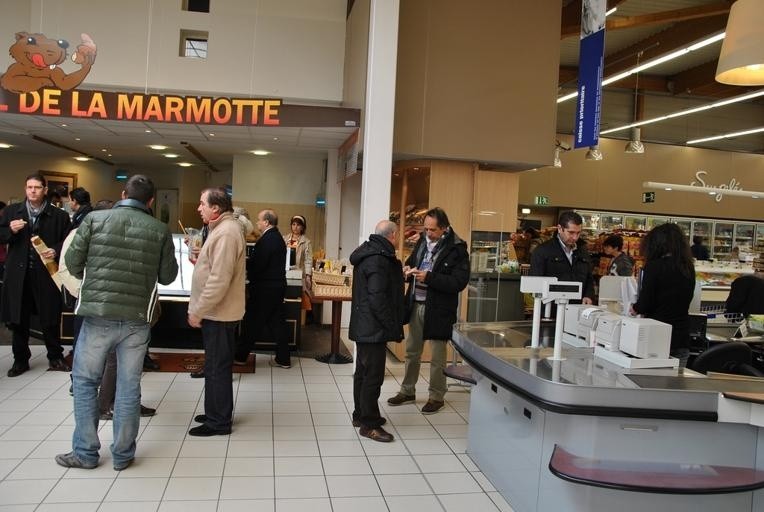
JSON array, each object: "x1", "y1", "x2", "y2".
[{"x1": 559, "y1": 140, "x2": 571, "y2": 150}]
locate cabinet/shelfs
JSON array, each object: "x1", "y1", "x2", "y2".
[{"x1": 559, "y1": 208, "x2": 764, "y2": 277}]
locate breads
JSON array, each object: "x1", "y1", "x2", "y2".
[{"x1": 30, "y1": 235, "x2": 57, "y2": 275}]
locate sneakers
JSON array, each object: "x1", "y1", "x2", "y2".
[
  {"x1": 388, "y1": 392, "x2": 416, "y2": 406},
  {"x1": 421, "y1": 401, "x2": 445, "y2": 415},
  {"x1": 140, "y1": 405, "x2": 156, "y2": 417},
  {"x1": 269, "y1": 359, "x2": 291, "y2": 368},
  {"x1": 55, "y1": 451, "x2": 97, "y2": 469},
  {"x1": 99, "y1": 407, "x2": 114, "y2": 420},
  {"x1": 114, "y1": 457, "x2": 135, "y2": 471},
  {"x1": 360, "y1": 424, "x2": 393, "y2": 442},
  {"x1": 352, "y1": 417, "x2": 386, "y2": 427},
  {"x1": 143, "y1": 355, "x2": 160, "y2": 370}
]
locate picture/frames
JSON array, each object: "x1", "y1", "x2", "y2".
[
  {"x1": 38, "y1": 170, "x2": 79, "y2": 202},
  {"x1": 154, "y1": 188, "x2": 180, "y2": 233}
]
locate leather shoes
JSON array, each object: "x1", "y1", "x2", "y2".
[
  {"x1": 190, "y1": 367, "x2": 205, "y2": 378},
  {"x1": 194, "y1": 415, "x2": 208, "y2": 423},
  {"x1": 8, "y1": 360, "x2": 29, "y2": 377},
  {"x1": 49, "y1": 357, "x2": 72, "y2": 372},
  {"x1": 189, "y1": 424, "x2": 231, "y2": 436}
]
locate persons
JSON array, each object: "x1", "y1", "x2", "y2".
[
  {"x1": 348, "y1": 219, "x2": 419, "y2": 443},
  {"x1": 513, "y1": 211, "x2": 764, "y2": 369},
  {"x1": 281, "y1": 214, "x2": 313, "y2": 290},
  {"x1": 180, "y1": 207, "x2": 253, "y2": 377},
  {"x1": 1, "y1": 173, "x2": 159, "y2": 421},
  {"x1": 57, "y1": 173, "x2": 179, "y2": 469},
  {"x1": 187, "y1": 186, "x2": 246, "y2": 436},
  {"x1": 230, "y1": 204, "x2": 262, "y2": 238},
  {"x1": 384, "y1": 206, "x2": 471, "y2": 414},
  {"x1": 229, "y1": 208, "x2": 291, "y2": 369}
]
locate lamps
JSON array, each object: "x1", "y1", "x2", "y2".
[
  {"x1": 553, "y1": 51, "x2": 645, "y2": 168},
  {"x1": 714, "y1": 1, "x2": 764, "y2": 86}
]
